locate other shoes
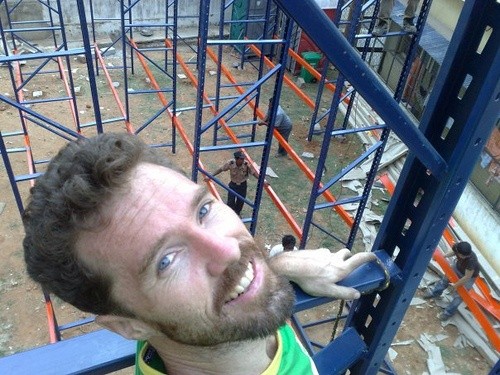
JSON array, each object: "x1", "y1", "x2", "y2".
[
  {"x1": 435, "y1": 311, "x2": 452, "y2": 321},
  {"x1": 419, "y1": 289, "x2": 441, "y2": 299},
  {"x1": 274, "y1": 151, "x2": 287, "y2": 158}
]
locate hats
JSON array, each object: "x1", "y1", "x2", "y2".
[{"x1": 233, "y1": 151, "x2": 246, "y2": 159}]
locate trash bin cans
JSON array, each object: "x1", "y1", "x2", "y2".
[{"x1": 301, "y1": 52, "x2": 322, "y2": 83}]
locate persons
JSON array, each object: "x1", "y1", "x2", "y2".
[
  {"x1": 371, "y1": 0, "x2": 420, "y2": 36},
  {"x1": 269, "y1": 235, "x2": 298, "y2": 258},
  {"x1": 21, "y1": 132, "x2": 377, "y2": 375},
  {"x1": 423, "y1": 241, "x2": 480, "y2": 321},
  {"x1": 203, "y1": 151, "x2": 270, "y2": 216},
  {"x1": 258, "y1": 97, "x2": 292, "y2": 157}
]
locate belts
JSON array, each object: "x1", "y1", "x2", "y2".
[{"x1": 231, "y1": 182, "x2": 246, "y2": 185}]
